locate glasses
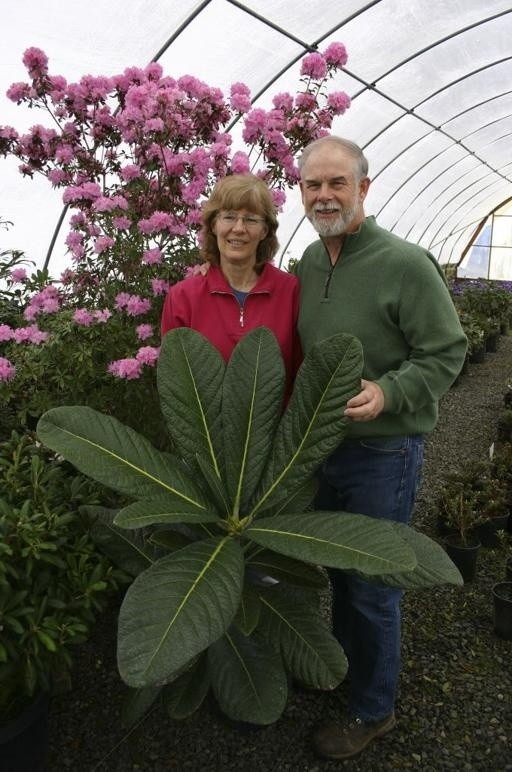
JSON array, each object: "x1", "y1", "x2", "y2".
[{"x1": 216, "y1": 213, "x2": 266, "y2": 226}]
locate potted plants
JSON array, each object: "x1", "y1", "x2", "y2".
[
  {"x1": 457, "y1": 286, "x2": 512, "y2": 363},
  {"x1": 434, "y1": 460, "x2": 512, "y2": 643}
]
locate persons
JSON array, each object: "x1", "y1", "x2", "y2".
[
  {"x1": 292, "y1": 137, "x2": 468, "y2": 759},
  {"x1": 161, "y1": 173, "x2": 305, "y2": 413}
]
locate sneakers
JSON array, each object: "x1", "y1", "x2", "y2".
[{"x1": 311, "y1": 708, "x2": 397, "y2": 760}]
